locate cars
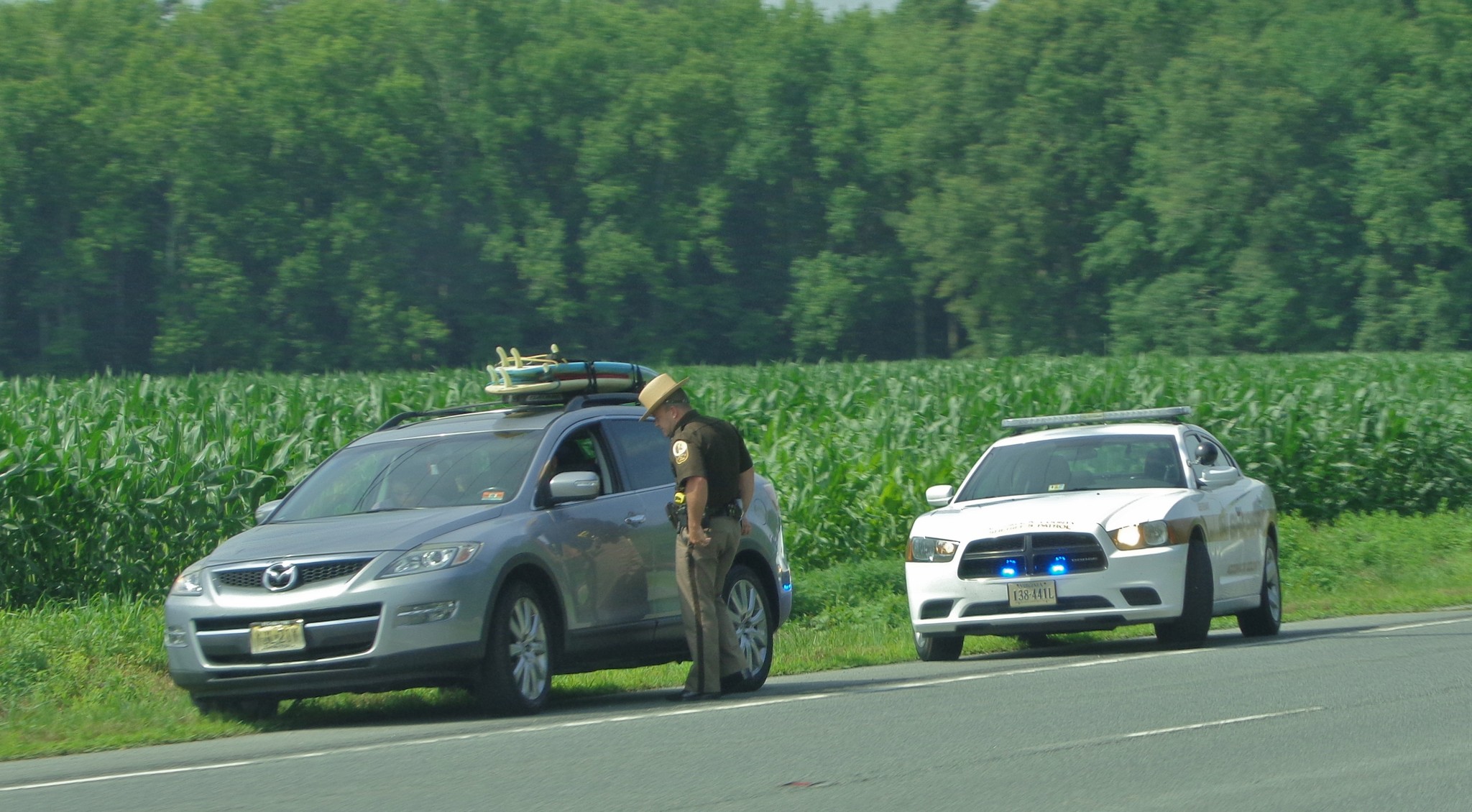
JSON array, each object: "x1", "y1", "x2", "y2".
[{"x1": 902, "y1": 406, "x2": 1282, "y2": 652}]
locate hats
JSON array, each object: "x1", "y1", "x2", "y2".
[{"x1": 637, "y1": 373, "x2": 690, "y2": 422}]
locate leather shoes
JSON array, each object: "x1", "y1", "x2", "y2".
[{"x1": 665, "y1": 670, "x2": 747, "y2": 701}]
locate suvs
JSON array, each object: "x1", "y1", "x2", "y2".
[{"x1": 156, "y1": 350, "x2": 795, "y2": 708}]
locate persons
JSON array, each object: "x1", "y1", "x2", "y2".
[
  {"x1": 636, "y1": 372, "x2": 755, "y2": 703},
  {"x1": 1026, "y1": 453, "x2": 1071, "y2": 491},
  {"x1": 371, "y1": 435, "x2": 569, "y2": 510}
]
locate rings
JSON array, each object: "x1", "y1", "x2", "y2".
[{"x1": 701, "y1": 543, "x2": 705, "y2": 546}]
